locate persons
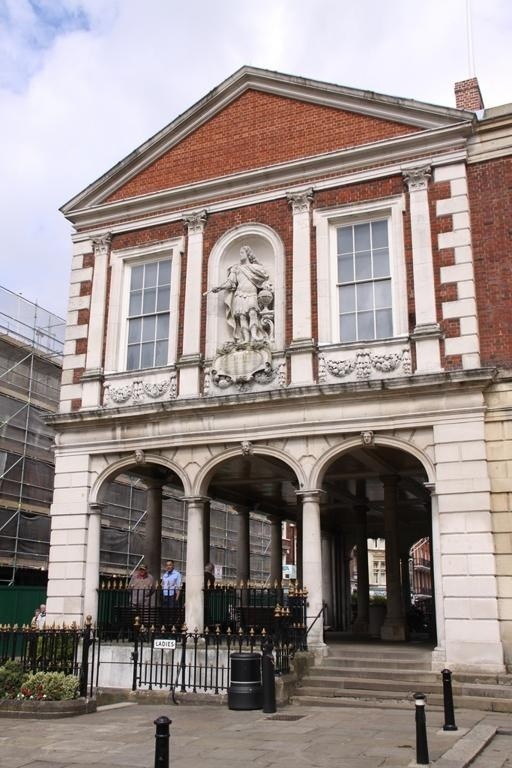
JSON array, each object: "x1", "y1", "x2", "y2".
[
  {"x1": 161, "y1": 559, "x2": 184, "y2": 640},
  {"x1": 34, "y1": 603, "x2": 47, "y2": 631},
  {"x1": 125, "y1": 562, "x2": 156, "y2": 643},
  {"x1": 212, "y1": 245, "x2": 270, "y2": 345},
  {"x1": 205, "y1": 563, "x2": 217, "y2": 630},
  {"x1": 30, "y1": 609, "x2": 41, "y2": 632}
]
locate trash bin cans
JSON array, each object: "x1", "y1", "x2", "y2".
[{"x1": 228, "y1": 653, "x2": 262, "y2": 711}]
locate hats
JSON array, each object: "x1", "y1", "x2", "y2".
[{"x1": 139, "y1": 565, "x2": 147, "y2": 569}]
